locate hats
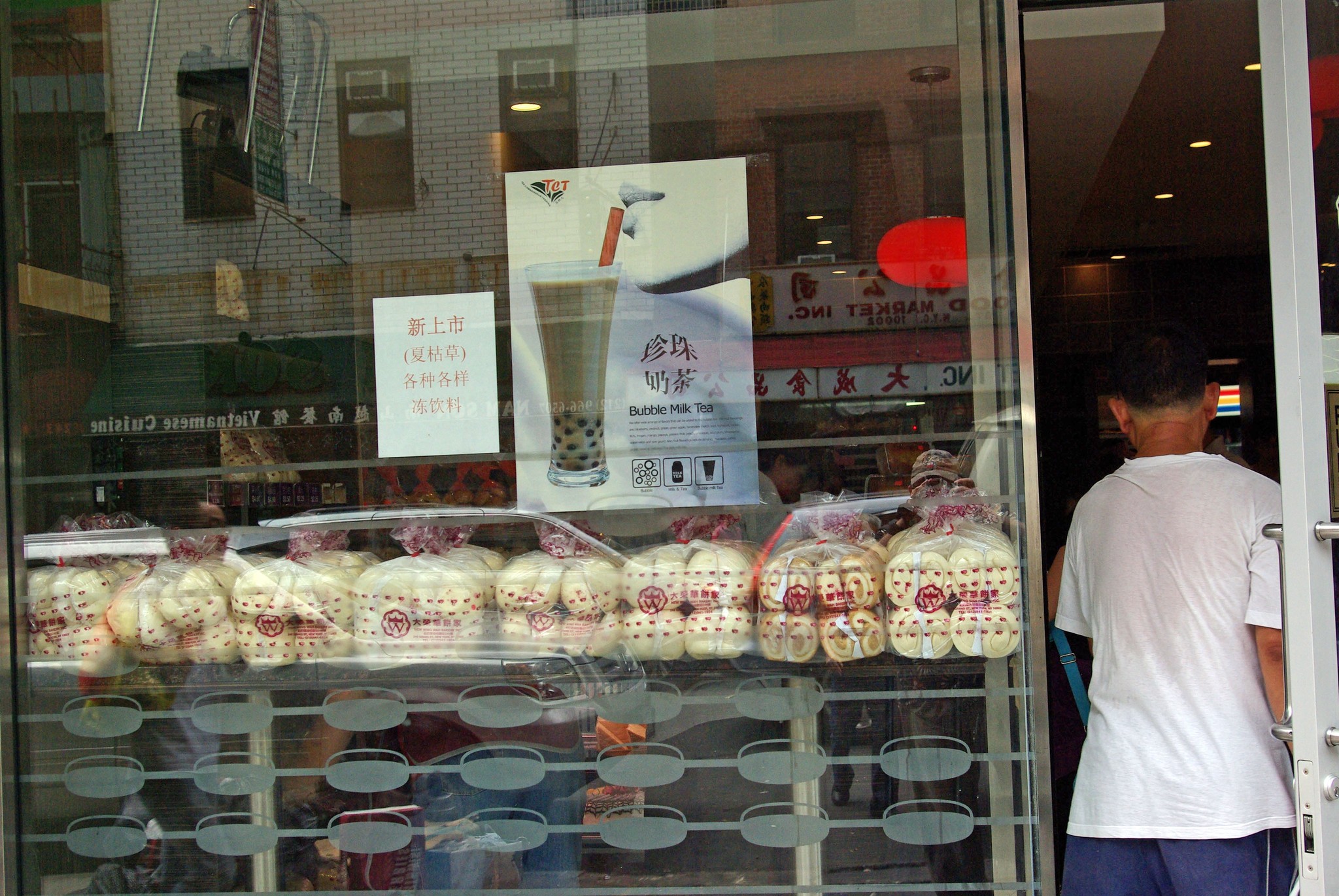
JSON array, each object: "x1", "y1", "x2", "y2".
[{"x1": 908, "y1": 448, "x2": 964, "y2": 491}]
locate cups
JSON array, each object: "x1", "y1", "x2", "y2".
[{"x1": 524, "y1": 257, "x2": 624, "y2": 489}]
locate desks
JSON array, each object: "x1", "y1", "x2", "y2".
[{"x1": 25, "y1": 657, "x2": 1016, "y2": 896}]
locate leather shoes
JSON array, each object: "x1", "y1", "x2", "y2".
[
  {"x1": 870, "y1": 789, "x2": 893, "y2": 818},
  {"x1": 831, "y1": 765, "x2": 856, "y2": 808}
]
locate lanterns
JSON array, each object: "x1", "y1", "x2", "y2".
[
  {"x1": 1308, "y1": 49, "x2": 1339, "y2": 151},
  {"x1": 876, "y1": 215, "x2": 970, "y2": 290}
]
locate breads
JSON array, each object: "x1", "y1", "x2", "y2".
[{"x1": 29, "y1": 518, "x2": 1023, "y2": 663}]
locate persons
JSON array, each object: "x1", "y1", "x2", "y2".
[
  {"x1": 126, "y1": 490, "x2": 229, "y2": 895},
  {"x1": 757, "y1": 449, "x2": 809, "y2": 506},
  {"x1": 826, "y1": 448, "x2": 1024, "y2": 895},
  {"x1": 1046, "y1": 323, "x2": 1299, "y2": 896},
  {"x1": 392, "y1": 681, "x2": 587, "y2": 890}
]
